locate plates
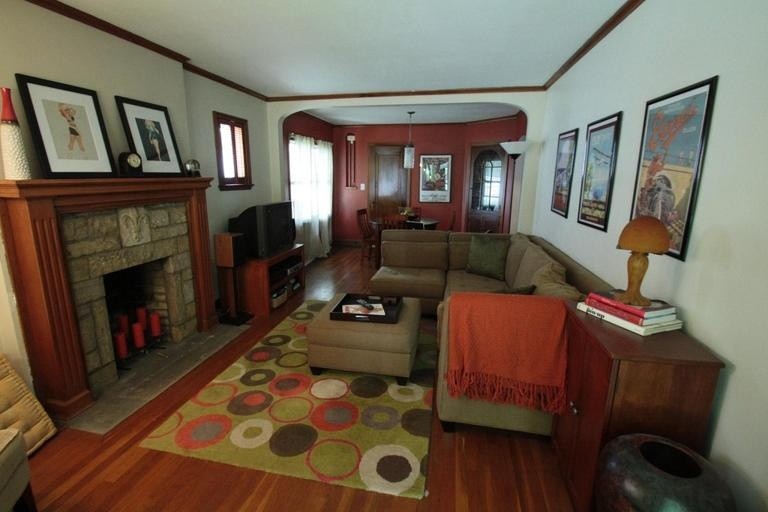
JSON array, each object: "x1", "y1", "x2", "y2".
[
  {"x1": 216, "y1": 242, "x2": 309, "y2": 317},
  {"x1": 546, "y1": 299, "x2": 729, "y2": 511}
]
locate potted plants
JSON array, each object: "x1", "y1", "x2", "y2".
[
  {"x1": 624, "y1": 72, "x2": 725, "y2": 264},
  {"x1": 416, "y1": 152, "x2": 454, "y2": 205},
  {"x1": 13, "y1": 68, "x2": 120, "y2": 179},
  {"x1": 112, "y1": 88, "x2": 187, "y2": 178},
  {"x1": 547, "y1": 126, "x2": 580, "y2": 220},
  {"x1": 573, "y1": 108, "x2": 624, "y2": 233}
]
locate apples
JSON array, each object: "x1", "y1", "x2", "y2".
[{"x1": 109, "y1": 304, "x2": 163, "y2": 361}]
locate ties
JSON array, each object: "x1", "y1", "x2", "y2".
[{"x1": 228, "y1": 200, "x2": 293, "y2": 258}]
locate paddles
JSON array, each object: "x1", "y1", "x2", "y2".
[{"x1": 136, "y1": 296, "x2": 440, "y2": 503}]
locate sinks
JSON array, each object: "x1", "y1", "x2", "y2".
[{"x1": 431, "y1": 226, "x2": 620, "y2": 445}]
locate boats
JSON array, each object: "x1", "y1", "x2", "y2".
[
  {"x1": 215, "y1": 231, "x2": 244, "y2": 268},
  {"x1": 292, "y1": 218, "x2": 296, "y2": 241}
]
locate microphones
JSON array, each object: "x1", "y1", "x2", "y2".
[{"x1": 0, "y1": 424, "x2": 41, "y2": 510}]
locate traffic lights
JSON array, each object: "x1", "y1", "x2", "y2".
[{"x1": 379, "y1": 233, "x2": 590, "y2": 302}]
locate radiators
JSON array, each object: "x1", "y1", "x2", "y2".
[{"x1": 357, "y1": 299, "x2": 373, "y2": 310}]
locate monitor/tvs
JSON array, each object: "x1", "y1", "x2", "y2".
[{"x1": 576, "y1": 289, "x2": 683, "y2": 337}]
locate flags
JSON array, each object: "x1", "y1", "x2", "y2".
[{"x1": 597, "y1": 430, "x2": 738, "y2": 511}]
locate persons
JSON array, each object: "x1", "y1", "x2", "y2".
[
  {"x1": 145, "y1": 119, "x2": 162, "y2": 161},
  {"x1": 60, "y1": 105, "x2": 87, "y2": 152}
]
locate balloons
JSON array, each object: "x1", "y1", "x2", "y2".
[{"x1": 115, "y1": 150, "x2": 144, "y2": 179}]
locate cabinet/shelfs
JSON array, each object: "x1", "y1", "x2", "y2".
[{"x1": 414, "y1": 202, "x2": 422, "y2": 220}]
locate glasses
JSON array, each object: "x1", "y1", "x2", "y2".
[
  {"x1": 613, "y1": 212, "x2": 673, "y2": 311},
  {"x1": 398, "y1": 108, "x2": 415, "y2": 170},
  {"x1": 497, "y1": 140, "x2": 531, "y2": 232}
]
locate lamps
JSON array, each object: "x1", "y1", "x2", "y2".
[
  {"x1": 368, "y1": 226, "x2": 451, "y2": 317},
  {"x1": 354, "y1": 199, "x2": 457, "y2": 272}
]
